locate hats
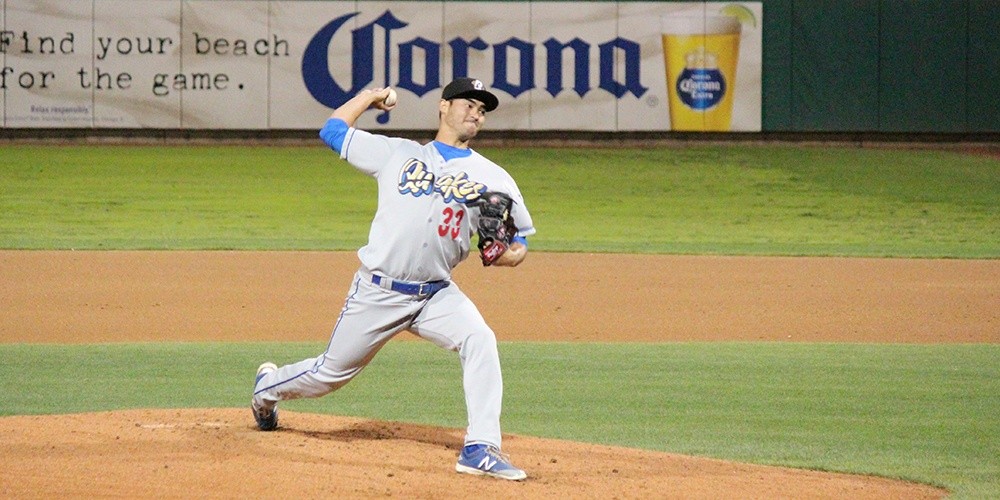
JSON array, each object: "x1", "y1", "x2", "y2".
[{"x1": 441, "y1": 78, "x2": 500, "y2": 112}]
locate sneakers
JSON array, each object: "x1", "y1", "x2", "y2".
[
  {"x1": 251, "y1": 362, "x2": 278, "y2": 431},
  {"x1": 454, "y1": 443, "x2": 528, "y2": 482}
]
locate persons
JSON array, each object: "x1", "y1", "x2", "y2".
[{"x1": 250, "y1": 76, "x2": 538, "y2": 482}]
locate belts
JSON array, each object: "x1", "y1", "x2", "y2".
[{"x1": 372, "y1": 274, "x2": 450, "y2": 297}]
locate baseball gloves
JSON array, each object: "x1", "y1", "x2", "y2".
[{"x1": 466, "y1": 190, "x2": 521, "y2": 267}]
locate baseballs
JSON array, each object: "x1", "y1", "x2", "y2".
[{"x1": 383, "y1": 88, "x2": 397, "y2": 107}]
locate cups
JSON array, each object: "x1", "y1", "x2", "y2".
[{"x1": 662, "y1": 12, "x2": 743, "y2": 129}]
